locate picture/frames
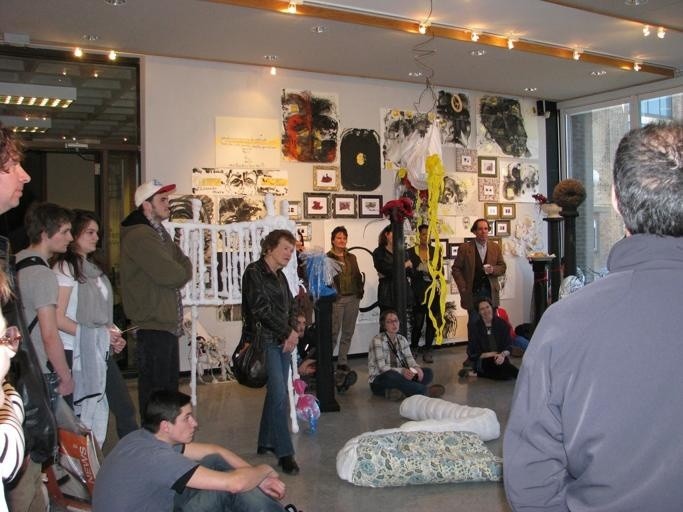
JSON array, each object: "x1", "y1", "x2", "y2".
[
  {"x1": 430, "y1": 147, "x2": 516, "y2": 293},
  {"x1": 286, "y1": 165, "x2": 383, "y2": 243}
]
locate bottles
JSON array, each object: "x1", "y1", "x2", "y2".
[{"x1": 307, "y1": 408, "x2": 317, "y2": 433}]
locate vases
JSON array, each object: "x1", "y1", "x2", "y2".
[{"x1": 539, "y1": 203, "x2": 563, "y2": 218}]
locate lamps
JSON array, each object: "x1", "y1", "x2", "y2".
[
  {"x1": 0, "y1": 83, "x2": 77, "y2": 111},
  {"x1": 0, "y1": 115, "x2": 52, "y2": 136}
]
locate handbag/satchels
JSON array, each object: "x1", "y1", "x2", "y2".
[{"x1": 232, "y1": 327, "x2": 267, "y2": 388}]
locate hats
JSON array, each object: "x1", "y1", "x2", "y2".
[{"x1": 134, "y1": 179, "x2": 176, "y2": 208}]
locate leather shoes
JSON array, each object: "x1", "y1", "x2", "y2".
[
  {"x1": 258, "y1": 447, "x2": 276, "y2": 453},
  {"x1": 278, "y1": 456, "x2": 299, "y2": 474}
]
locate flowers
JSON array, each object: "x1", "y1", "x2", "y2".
[{"x1": 531, "y1": 193, "x2": 548, "y2": 204}]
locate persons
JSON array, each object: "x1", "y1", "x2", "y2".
[
  {"x1": 1, "y1": 129, "x2": 286, "y2": 512},
  {"x1": 242, "y1": 227, "x2": 365, "y2": 474},
  {"x1": 500, "y1": 116, "x2": 682, "y2": 512},
  {"x1": 370, "y1": 219, "x2": 529, "y2": 401}
]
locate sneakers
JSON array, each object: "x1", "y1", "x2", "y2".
[
  {"x1": 427, "y1": 384, "x2": 445, "y2": 397},
  {"x1": 422, "y1": 351, "x2": 433, "y2": 363},
  {"x1": 384, "y1": 388, "x2": 402, "y2": 400},
  {"x1": 512, "y1": 349, "x2": 523, "y2": 356},
  {"x1": 463, "y1": 358, "x2": 471, "y2": 366},
  {"x1": 337, "y1": 363, "x2": 351, "y2": 372},
  {"x1": 337, "y1": 372, "x2": 357, "y2": 394},
  {"x1": 459, "y1": 369, "x2": 474, "y2": 377}
]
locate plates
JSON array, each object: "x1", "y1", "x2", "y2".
[{"x1": 525, "y1": 254, "x2": 558, "y2": 262}]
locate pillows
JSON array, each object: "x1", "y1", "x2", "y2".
[{"x1": 352, "y1": 430, "x2": 503, "y2": 489}]
